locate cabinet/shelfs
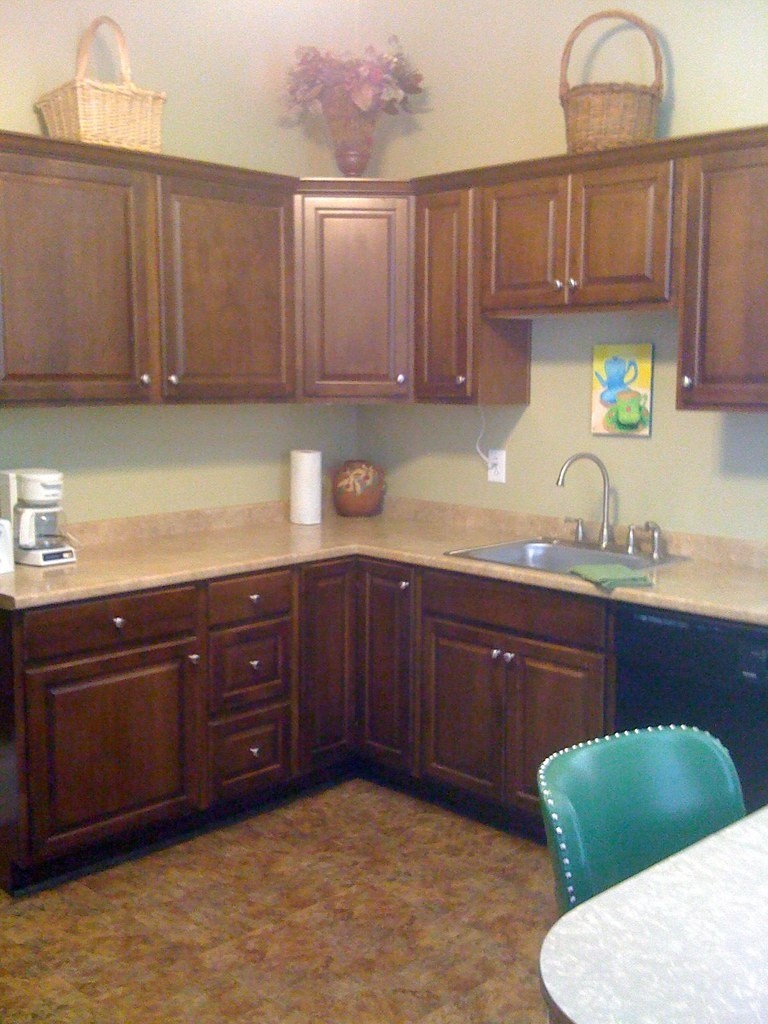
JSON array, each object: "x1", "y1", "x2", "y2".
[
  {"x1": 413, "y1": 169, "x2": 532, "y2": 408},
  {"x1": 3, "y1": 586, "x2": 204, "y2": 895},
  {"x1": 297, "y1": 175, "x2": 418, "y2": 405},
  {"x1": 676, "y1": 124, "x2": 768, "y2": 413},
  {"x1": 420, "y1": 565, "x2": 617, "y2": 837},
  {"x1": 208, "y1": 567, "x2": 292, "y2": 813},
  {"x1": 355, "y1": 558, "x2": 418, "y2": 778},
  {"x1": 0, "y1": 130, "x2": 296, "y2": 406},
  {"x1": 476, "y1": 141, "x2": 675, "y2": 317}
]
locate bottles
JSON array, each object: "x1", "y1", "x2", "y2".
[{"x1": 333, "y1": 460, "x2": 382, "y2": 516}]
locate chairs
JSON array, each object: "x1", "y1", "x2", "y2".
[{"x1": 538, "y1": 723, "x2": 747, "y2": 917}]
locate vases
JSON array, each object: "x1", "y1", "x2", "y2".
[{"x1": 332, "y1": 132, "x2": 372, "y2": 178}]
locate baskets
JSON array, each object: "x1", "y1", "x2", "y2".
[
  {"x1": 560, "y1": 8, "x2": 663, "y2": 158},
  {"x1": 32, "y1": 14, "x2": 166, "y2": 153}
]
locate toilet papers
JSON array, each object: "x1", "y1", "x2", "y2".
[{"x1": 289, "y1": 449, "x2": 323, "y2": 525}]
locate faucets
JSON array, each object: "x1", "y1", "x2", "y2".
[
  {"x1": 644, "y1": 521, "x2": 663, "y2": 560},
  {"x1": 556, "y1": 452, "x2": 642, "y2": 555}
]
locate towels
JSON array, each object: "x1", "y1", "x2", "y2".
[{"x1": 566, "y1": 564, "x2": 656, "y2": 592}]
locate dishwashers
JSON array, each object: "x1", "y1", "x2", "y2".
[{"x1": 615, "y1": 607, "x2": 768, "y2": 813}]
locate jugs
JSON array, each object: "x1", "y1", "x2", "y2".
[{"x1": 12, "y1": 501, "x2": 69, "y2": 551}]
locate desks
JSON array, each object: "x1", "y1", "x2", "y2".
[{"x1": 540, "y1": 805, "x2": 768, "y2": 1024}]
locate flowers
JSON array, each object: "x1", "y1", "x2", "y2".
[{"x1": 272, "y1": 30, "x2": 424, "y2": 148}]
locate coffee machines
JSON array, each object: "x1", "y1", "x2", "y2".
[{"x1": 0, "y1": 467, "x2": 76, "y2": 566}]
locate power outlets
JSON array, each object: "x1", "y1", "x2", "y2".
[{"x1": 487, "y1": 450, "x2": 506, "y2": 483}]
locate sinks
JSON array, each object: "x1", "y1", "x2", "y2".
[{"x1": 442, "y1": 536, "x2": 692, "y2": 577}]
